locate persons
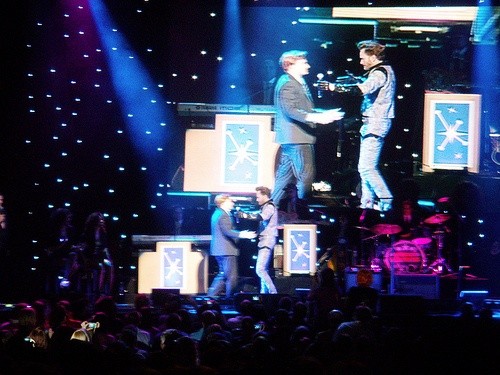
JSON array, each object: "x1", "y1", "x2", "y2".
[
  {"x1": 0, "y1": 294, "x2": 372, "y2": 375},
  {"x1": 207, "y1": 194, "x2": 258, "y2": 299},
  {"x1": 313, "y1": 40, "x2": 396, "y2": 212},
  {"x1": 394, "y1": 197, "x2": 423, "y2": 240},
  {"x1": 234, "y1": 186, "x2": 279, "y2": 294},
  {"x1": 270, "y1": 50, "x2": 345, "y2": 220},
  {"x1": 0, "y1": 195, "x2": 6, "y2": 229},
  {"x1": 277, "y1": 181, "x2": 311, "y2": 221},
  {"x1": 160, "y1": 204, "x2": 197, "y2": 236},
  {"x1": 38, "y1": 209, "x2": 115, "y2": 298}
]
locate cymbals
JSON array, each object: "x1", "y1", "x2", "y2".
[
  {"x1": 371, "y1": 223, "x2": 402, "y2": 235},
  {"x1": 412, "y1": 238, "x2": 431, "y2": 244},
  {"x1": 438, "y1": 196, "x2": 450, "y2": 203},
  {"x1": 425, "y1": 214, "x2": 450, "y2": 224},
  {"x1": 354, "y1": 226, "x2": 369, "y2": 230}
]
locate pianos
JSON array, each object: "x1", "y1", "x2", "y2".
[
  {"x1": 132, "y1": 234, "x2": 212, "y2": 246},
  {"x1": 177, "y1": 102, "x2": 276, "y2": 119}
]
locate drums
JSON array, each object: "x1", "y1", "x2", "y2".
[{"x1": 376, "y1": 240, "x2": 425, "y2": 273}]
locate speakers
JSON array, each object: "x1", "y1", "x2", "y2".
[
  {"x1": 377, "y1": 294, "x2": 428, "y2": 327},
  {"x1": 346, "y1": 273, "x2": 381, "y2": 293},
  {"x1": 389, "y1": 274, "x2": 440, "y2": 299}
]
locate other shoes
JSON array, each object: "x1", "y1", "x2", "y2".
[
  {"x1": 380, "y1": 199, "x2": 393, "y2": 211},
  {"x1": 360, "y1": 203, "x2": 373, "y2": 209}
]
